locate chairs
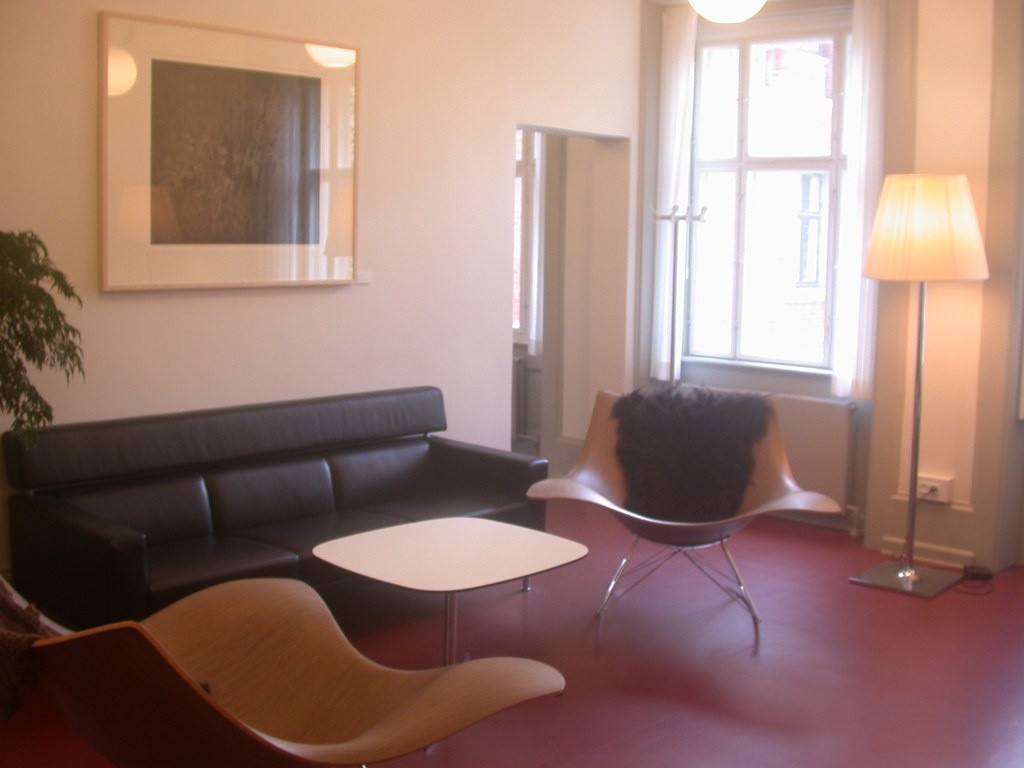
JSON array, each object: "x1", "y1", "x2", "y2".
[
  {"x1": 0, "y1": 570, "x2": 566, "y2": 768},
  {"x1": 526, "y1": 388, "x2": 847, "y2": 621}
]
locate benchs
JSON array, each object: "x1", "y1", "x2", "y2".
[{"x1": 0, "y1": 385, "x2": 551, "y2": 634}]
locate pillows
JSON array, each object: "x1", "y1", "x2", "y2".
[{"x1": 610, "y1": 386, "x2": 777, "y2": 524}]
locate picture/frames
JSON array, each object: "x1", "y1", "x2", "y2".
[{"x1": 96, "y1": 10, "x2": 358, "y2": 292}]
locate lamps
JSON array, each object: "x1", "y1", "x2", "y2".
[{"x1": 848, "y1": 172, "x2": 990, "y2": 601}]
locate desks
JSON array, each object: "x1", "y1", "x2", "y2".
[{"x1": 310, "y1": 516, "x2": 589, "y2": 753}]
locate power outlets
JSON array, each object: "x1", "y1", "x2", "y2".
[{"x1": 917, "y1": 473, "x2": 954, "y2": 504}]
